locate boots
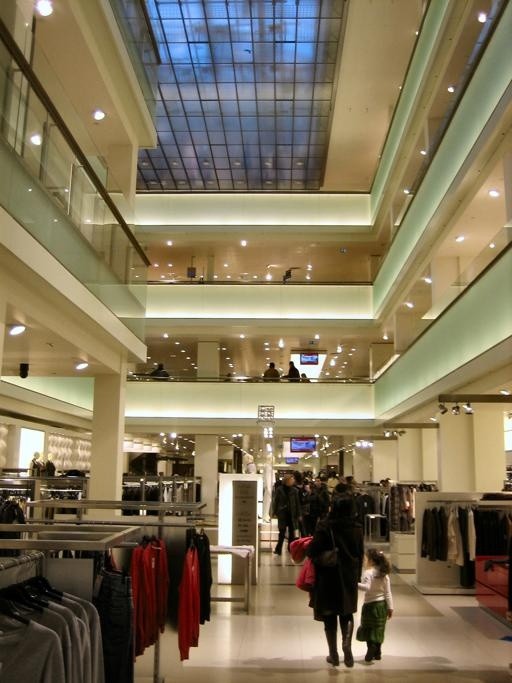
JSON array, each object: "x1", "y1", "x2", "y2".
[
  {"x1": 324, "y1": 628, "x2": 339, "y2": 666},
  {"x1": 342, "y1": 620, "x2": 354, "y2": 667}
]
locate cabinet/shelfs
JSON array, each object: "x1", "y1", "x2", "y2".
[
  {"x1": 0, "y1": 519, "x2": 205, "y2": 625},
  {"x1": 426, "y1": 499, "x2": 476, "y2": 512}
]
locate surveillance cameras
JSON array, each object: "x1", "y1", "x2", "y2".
[{"x1": 19, "y1": 363, "x2": 28, "y2": 378}]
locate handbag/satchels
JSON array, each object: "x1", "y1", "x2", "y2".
[{"x1": 313, "y1": 546, "x2": 341, "y2": 582}]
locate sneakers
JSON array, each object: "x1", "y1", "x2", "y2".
[{"x1": 365, "y1": 644, "x2": 381, "y2": 661}]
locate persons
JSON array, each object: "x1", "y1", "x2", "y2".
[
  {"x1": 281, "y1": 360, "x2": 300, "y2": 381},
  {"x1": 270, "y1": 465, "x2": 356, "y2": 554},
  {"x1": 149, "y1": 362, "x2": 170, "y2": 381},
  {"x1": 300, "y1": 372, "x2": 311, "y2": 382},
  {"x1": 307, "y1": 490, "x2": 365, "y2": 667},
  {"x1": 44, "y1": 452, "x2": 56, "y2": 475},
  {"x1": 29, "y1": 451, "x2": 42, "y2": 477},
  {"x1": 354, "y1": 544, "x2": 392, "y2": 661},
  {"x1": 222, "y1": 372, "x2": 233, "y2": 381},
  {"x1": 264, "y1": 361, "x2": 280, "y2": 382}
]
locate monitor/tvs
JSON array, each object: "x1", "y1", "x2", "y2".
[
  {"x1": 290, "y1": 437, "x2": 316, "y2": 453},
  {"x1": 285, "y1": 456, "x2": 299, "y2": 464},
  {"x1": 299, "y1": 353, "x2": 319, "y2": 365}
]
